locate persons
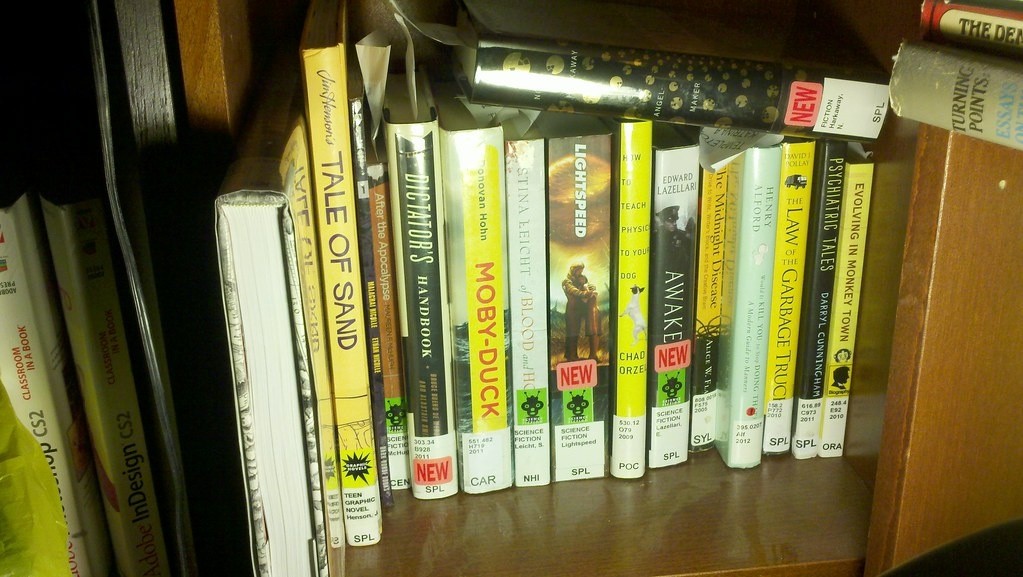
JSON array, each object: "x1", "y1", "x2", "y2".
[
  {"x1": 657, "y1": 205, "x2": 690, "y2": 271},
  {"x1": 676, "y1": 216, "x2": 696, "y2": 243}
]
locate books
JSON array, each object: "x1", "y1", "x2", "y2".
[{"x1": 0, "y1": 0, "x2": 1023, "y2": 577}]
